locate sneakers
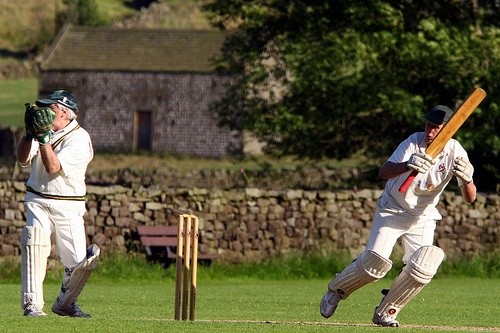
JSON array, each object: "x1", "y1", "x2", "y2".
[
  {"x1": 320, "y1": 290, "x2": 340, "y2": 318},
  {"x1": 372, "y1": 306, "x2": 399, "y2": 327},
  {"x1": 23, "y1": 302, "x2": 47, "y2": 316},
  {"x1": 51, "y1": 302, "x2": 91, "y2": 318}
]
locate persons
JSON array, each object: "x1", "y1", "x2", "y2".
[
  {"x1": 16, "y1": 89, "x2": 93, "y2": 318},
  {"x1": 319, "y1": 105, "x2": 477, "y2": 326}
]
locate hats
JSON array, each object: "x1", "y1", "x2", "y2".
[
  {"x1": 35, "y1": 90, "x2": 79, "y2": 112},
  {"x1": 421, "y1": 105, "x2": 453, "y2": 125}
]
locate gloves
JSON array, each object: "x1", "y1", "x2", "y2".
[
  {"x1": 406, "y1": 152, "x2": 436, "y2": 174},
  {"x1": 32, "y1": 107, "x2": 55, "y2": 144},
  {"x1": 23, "y1": 103, "x2": 38, "y2": 141},
  {"x1": 452, "y1": 157, "x2": 474, "y2": 183}
]
